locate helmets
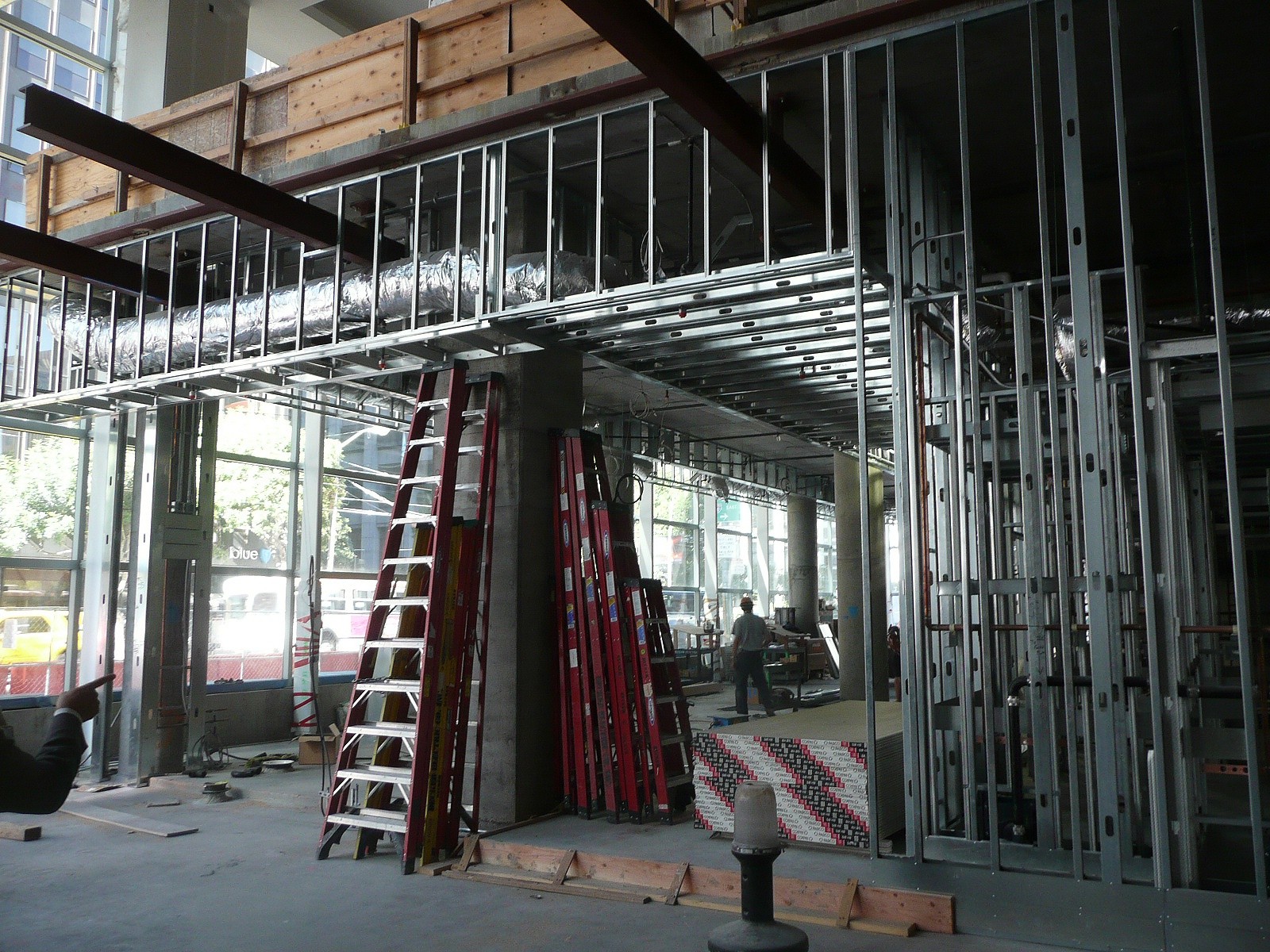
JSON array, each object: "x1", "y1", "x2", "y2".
[{"x1": 740, "y1": 597, "x2": 754, "y2": 605}]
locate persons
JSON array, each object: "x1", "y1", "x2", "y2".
[
  {"x1": 0, "y1": 674, "x2": 117, "y2": 815},
  {"x1": 729, "y1": 596, "x2": 777, "y2": 722}
]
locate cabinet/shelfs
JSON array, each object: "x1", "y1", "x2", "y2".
[
  {"x1": 747, "y1": 635, "x2": 804, "y2": 687},
  {"x1": 804, "y1": 638, "x2": 827, "y2": 681}
]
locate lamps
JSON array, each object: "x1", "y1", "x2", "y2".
[
  {"x1": 665, "y1": 391, "x2": 669, "y2": 401},
  {"x1": 776, "y1": 434, "x2": 781, "y2": 442}
]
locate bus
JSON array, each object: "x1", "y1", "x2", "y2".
[
  {"x1": 0, "y1": 558, "x2": 283, "y2": 695},
  {"x1": 223, "y1": 569, "x2": 406, "y2": 671}
]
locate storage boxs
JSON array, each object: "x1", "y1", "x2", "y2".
[{"x1": 287, "y1": 723, "x2": 341, "y2": 765}]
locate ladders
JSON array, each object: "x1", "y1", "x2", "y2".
[
  {"x1": 316, "y1": 362, "x2": 508, "y2": 877},
  {"x1": 545, "y1": 425, "x2": 699, "y2": 828}
]
